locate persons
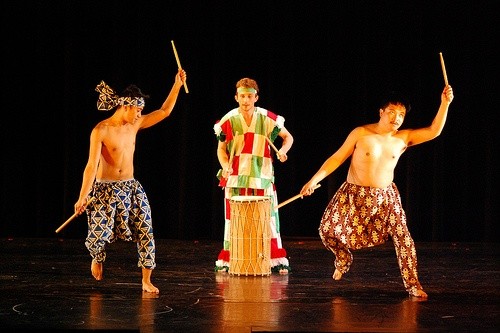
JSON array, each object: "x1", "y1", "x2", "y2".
[
  {"x1": 300, "y1": 83, "x2": 455, "y2": 299},
  {"x1": 73, "y1": 69, "x2": 186, "y2": 294},
  {"x1": 213, "y1": 78, "x2": 295, "y2": 277}
]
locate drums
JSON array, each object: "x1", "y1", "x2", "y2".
[{"x1": 228, "y1": 196, "x2": 272, "y2": 276}]
